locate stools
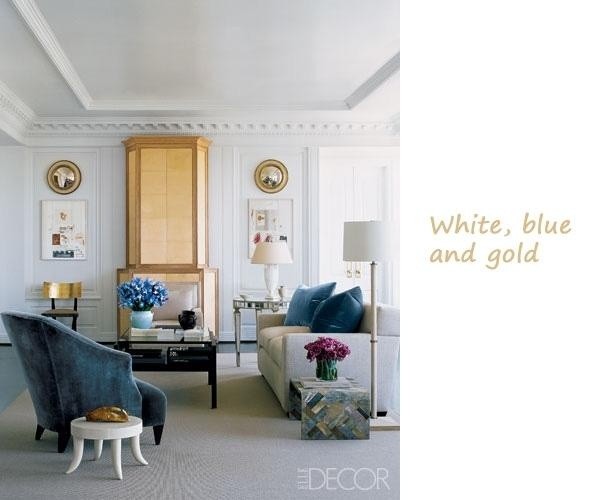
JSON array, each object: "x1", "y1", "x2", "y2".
[{"x1": 64, "y1": 416, "x2": 149, "y2": 480}]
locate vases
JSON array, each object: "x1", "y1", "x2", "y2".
[
  {"x1": 316, "y1": 360, "x2": 338, "y2": 382},
  {"x1": 131, "y1": 311, "x2": 153, "y2": 329}
]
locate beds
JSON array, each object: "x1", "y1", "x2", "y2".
[{"x1": 113, "y1": 325, "x2": 218, "y2": 410}]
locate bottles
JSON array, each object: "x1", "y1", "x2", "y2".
[{"x1": 178, "y1": 310, "x2": 197, "y2": 330}]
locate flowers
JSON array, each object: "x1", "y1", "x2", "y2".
[
  {"x1": 303, "y1": 337, "x2": 352, "y2": 362},
  {"x1": 116, "y1": 277, "x2": 169, "y2": 311}
]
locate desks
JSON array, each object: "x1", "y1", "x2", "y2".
[
  {"x1": 232, "y1": 295, "x2": 290, "y2": 368},
  {"x1": 287, "y1": 376, "x2": 371, "y2": 441}
]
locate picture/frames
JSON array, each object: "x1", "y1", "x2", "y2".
[
  {"x1": 254, "y1": 159, "x2": 289, "y2": 194},
  {"x1": 48, "y1": 160, "x2": 82, "y2": 195},
  {"x1": 40, "y1": 198, "x2": 88, "y2": 262},
  {"x1": 247, "y1": 196, "x2": 294, "y2": 260}
]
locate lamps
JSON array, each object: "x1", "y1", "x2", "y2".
[
  {"x1": 342, "y1": 219, "x2": 400, "y2": 427},
  {"x1": 251, "y1": 241, "x2": 294, "y2": 298}
]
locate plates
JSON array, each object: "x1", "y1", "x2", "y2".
[{"x1": 240, "y1": 294, "x2": 253, "y2": 299}]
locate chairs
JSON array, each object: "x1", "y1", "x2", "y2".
[
  {"x1": 0, "y1": 310, "x2": 168, "y2": 454},
  {"x1": 41, "y1": 280, "x2": 82, "y2": 333}
]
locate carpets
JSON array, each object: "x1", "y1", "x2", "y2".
[{"x1": 0, "y1": 352, "x2": 400, "y2": 500}]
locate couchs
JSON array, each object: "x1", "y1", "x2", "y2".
[{"x1": 256, "y1": 302, "x2": 399, "y2": 417}]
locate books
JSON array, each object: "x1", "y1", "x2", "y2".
[
  {"x1": 131, "y1": 328, "x2": 162, "y2": 337},
  {"x1": 299, "y1": 376, "x2": 350, "y2": 389}
]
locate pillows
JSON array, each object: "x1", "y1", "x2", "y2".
[
  {"x1": 282, "y1": 281, "x2": 337, "y2": 326},
  {"x1": 309, "y1": 286, "x2": 365, "y2": 333}
]
